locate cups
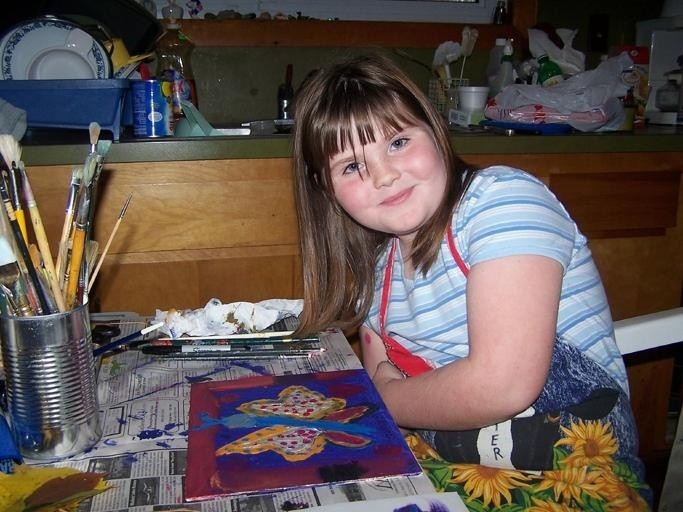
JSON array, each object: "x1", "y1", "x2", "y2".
[
  {"x1": 458, "y1": 86, "x2": 490, "y2": 114},
  {"x1": 0, "y1": 304, "x2": 105, "y2": 465}
]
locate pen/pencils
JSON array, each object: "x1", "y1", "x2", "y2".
[
  {"x1": 130, "y1": 332, "x2": 328, "y2": 361},
  {"x1": 92, "y1": 320, "x2": 165, "y2": 357}
]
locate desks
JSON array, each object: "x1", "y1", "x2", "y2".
[
  {"x1": 0, "y1": 310, "x2": 435, "y2": 512},
  {"x1": 18, "y1": 130, "x2": 683, "y2": 511}
]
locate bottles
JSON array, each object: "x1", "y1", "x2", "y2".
[
  {"x1": 498, "y1": 39, "x2": 517, "y2": 90},
  {"x1": 275, "y1": 82, "x2": 295, "y2": 134},
  {"x1": 533, "y1": 48, "x2": 565, "y2": 87},
  {"x1": 151, "y1": 4, "x2": 198, "y2": 113},
  {"x1": 484, "y1": 38, "x2": 507, "y2": 87},
  {"x1": 493, "y1": 0, "x2": 508, "y2": 25}
]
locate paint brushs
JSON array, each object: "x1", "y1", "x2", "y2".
[{"x1": 0, "y1": 122, "x2": 133, "y2": 318}]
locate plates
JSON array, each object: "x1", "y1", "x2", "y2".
[
  {"x1": 0, "y1": 16, "x2": 117, "y2": 81},
  {"x1": 113, "y1": 61, "x2": 143, "y2": 81}
]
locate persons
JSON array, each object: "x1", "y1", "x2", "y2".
[{"x1": 290, "y1": 50, "x2": 656, "y2": 512}]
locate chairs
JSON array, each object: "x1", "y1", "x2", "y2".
[{"x1": 614, "y1": 307, "x2": 683, "y2": 512}]
